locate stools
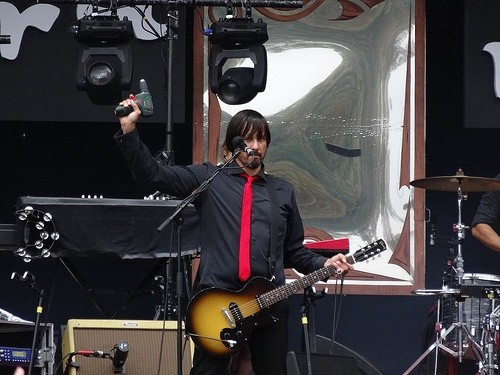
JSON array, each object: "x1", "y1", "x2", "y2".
[{"x1": 441, "y1": 343, "x2": 486, "y2": 375}]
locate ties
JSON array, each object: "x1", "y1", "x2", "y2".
[{"x1": 238, "y1": 172, "x2": 260, "y2": 281}]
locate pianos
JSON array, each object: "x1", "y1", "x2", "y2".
[{"x1": 301, "y1": 236, "x2": 350, "y2": 254}]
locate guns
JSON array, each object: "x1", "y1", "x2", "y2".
[{"x1": 115, "y1": 74, "x2": 156, "y2": 119}]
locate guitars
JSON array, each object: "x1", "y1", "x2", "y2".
[{"x1": 188, "y1": 239, "x2": 386, "y2": 354}]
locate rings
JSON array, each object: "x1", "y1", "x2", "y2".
[{"x1": 336, "y1": 269, "x2": 341, "y2": 274}]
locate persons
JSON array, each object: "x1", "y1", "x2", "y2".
[
  {"x1": 189, "y1": 142, "x2": 237, "y2": 288},
  {"x1": 471, "y1": 173, "x2": 500, "y2": 251},
  {"x1": 115, "y1": 94, "x2": 355, "y2": 375}
]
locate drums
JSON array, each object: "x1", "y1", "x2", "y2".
[{"x1": 441, "y1": 270, "x2": 500, "y2": 353}]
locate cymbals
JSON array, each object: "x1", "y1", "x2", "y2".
[
  {"x1": 409, "y1": 176, "x2": 500, "y2": 192},
  {"x1": 13, "y1": 205, "x2": 61, "y2": 264}
]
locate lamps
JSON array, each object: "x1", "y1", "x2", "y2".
[
  {"x1": 68, "y1": 0, "x2": 137, "y2": 103},
  {"x1": 202, "y1": 1, "x2": 269, "y2": 105}
]
